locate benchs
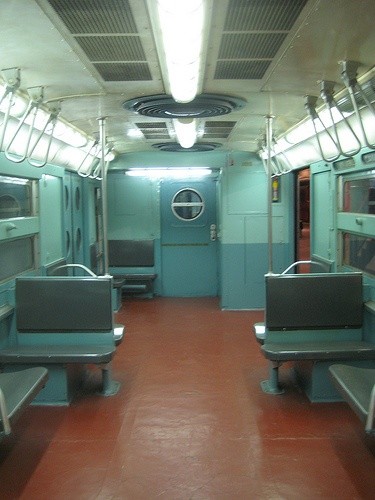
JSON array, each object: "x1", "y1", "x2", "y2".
[
  {"x1": 11, "y1": 273, "x2": 116, "y2": 396},
  {"x1": 99, "y1": 239, "x2": 158, "y2": 295},
  {"x1": 330, "y1": 356, "x2": 375, "y2": 440},
  {"x1": 260, "y1": 271, "x2": 375, "y2": 394},
  {"x1": 0, "y1": 363, "x2": 50, "y2": 438}
]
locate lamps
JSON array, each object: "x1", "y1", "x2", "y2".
[
  {"x1": 259, "y1": 136, "x2": 294, "y2": 160},
  {"x1": 155, "y1": 0, "x2": 204, "y2": 106},
  {"x1": 79, "y1": 138, "x2": 115, "y2": 163},
  {"x1": 285, "y1": 101, "x2": 374, "y2": 144},
  {"x1": 0, "y1": 85, "x2": 86, "y2": 149},
  {"x1": 172, "y1": 117, "x2": 197, "y2": 149}
]
